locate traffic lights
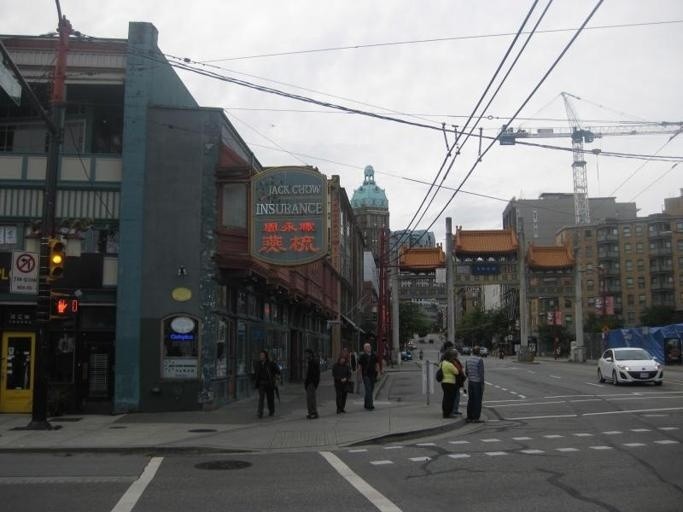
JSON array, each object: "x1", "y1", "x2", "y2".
[
  {"x1": 49, "y1": 293, "x2": 73, "y2": 318},
  {"x1": 47, "y1": 239, "x2": 64, "y2": 280}
]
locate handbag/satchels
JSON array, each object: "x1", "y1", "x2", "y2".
[
  {"x1": 436, "y1": 369, "x2": 443, "y2": 382},
  {"x1": 345, "y1": 381, "x2": 355, "y2": 393},
  {"x1": 455, "y1": 372, "x2": 467, "y2": 384}
]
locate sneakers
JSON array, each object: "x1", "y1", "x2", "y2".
[
  {"x1": 443, "y1": 410, "x2": 463, "y2": 418},
  {"x1": 364, "y1": 406, "x2": 374, "y2": 409},
  {"x1": 307, "y1": 414, "x2": 318, "y2": 419},
  {"x1": 256, "y1": 411, "x2": 275, "y2": 418},
  {"x1": 337, "y1": 408, "x2": 346, "y2": 414},
  {"x1": 466, "y1": 418, "x2": 485, "y2": 423}
]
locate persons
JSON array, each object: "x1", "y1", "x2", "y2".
[
  {"x1": 253, "y1": 350, "x2": 275, "y2": 417},
  {"x1": 357, "y1": 343, "x2": 380, "y2": 409},
  {"x1": 331, "y1": 355, "x2": 351, "y2": 414},
  {"x1": 340, "y1": 346, "x2": 352, "y2": 370},
  {"x1": 499, "y1": 347, "x2": 505, "y2": 359},
  {"x1": 303, "y1": 347, "x2": 320, "y2": 419},
  {"x1": 348, "y1": 351, "x2": 356, "y2": 371},
  {"x1": 442, "y1": 340, "x2": 467, "y2": 415},
  {"x1": 419, "y1": 349, "x2": 423, "y2": 360},
  {"x1": 464, "y1": 344, "x2": 485, "y2": 422},
  {"x1": 551, "y1": 336, "x2": 560, "y2": 360},
  {"x1": 436, "y1": 348, "x2": 460, "y2": 418}
]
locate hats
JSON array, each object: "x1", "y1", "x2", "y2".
[{"x1": 447, "y1": 348, "x2": 458, "y2": 361}]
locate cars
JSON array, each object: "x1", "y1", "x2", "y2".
[
  {"x1": 596, "y1": 347, "x2": 663, "y2": 387},
  {"x1": 399, "y1": 337, "x2": 490, "y2": 362}
]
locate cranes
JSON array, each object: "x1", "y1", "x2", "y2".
[{"x1": 497, "y1": 90, "x2": 682, "y2": 224}]
